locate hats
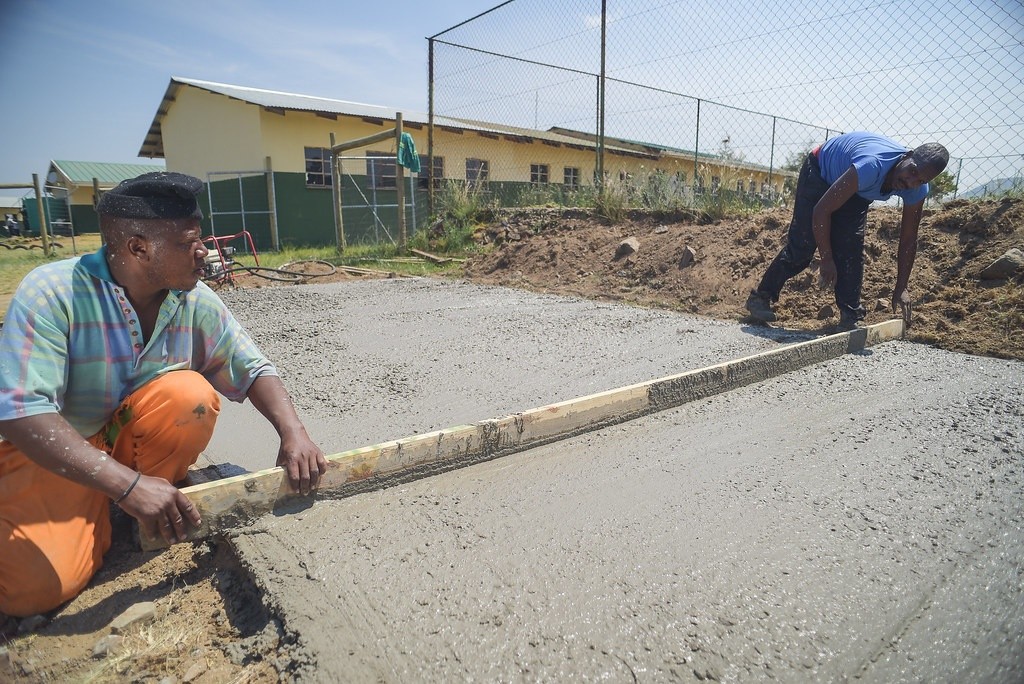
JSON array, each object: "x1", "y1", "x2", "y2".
[{"x1": 98, "y1": 171, "x2": 206, "y2": 220}]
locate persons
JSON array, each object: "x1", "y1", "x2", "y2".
[
  {"x1": 1, "y1": 172, "x2": 329, "y2": 617},
  {"x1": 744, "y1": 132, "x2": 949, "y2": 325},
  {"x1": 5, "y1": 204, "x2": 30, "y2": 237}
]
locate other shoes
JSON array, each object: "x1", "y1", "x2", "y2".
[
  {"x1": 745, "y1": 290, "x2": 778, "y2": 322},
  {"x1": 839, "y1": 310, "x2": 867, "y2": 330}
]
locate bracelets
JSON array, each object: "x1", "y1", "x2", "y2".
[{"x1": 114, "y1": 472, "x2": 144, "y2": 505}]
